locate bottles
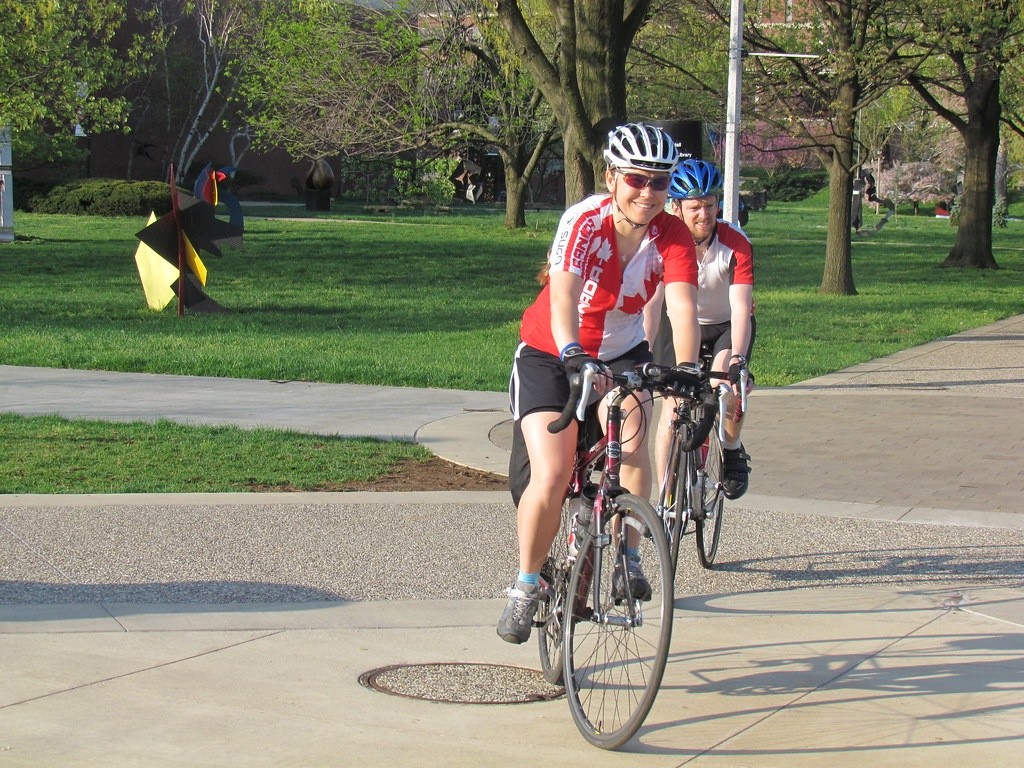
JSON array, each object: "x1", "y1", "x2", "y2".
[
  {"x1": 569, "y1": 492, "x2": 582, "y2": 556},
  {"x1": 691, "y1": 421, "x2": 703, "y2": 467},
  {"x1": 696, "y1": 417, "x2": 709, "y2": 469},
  {"x1": 575, "y1": 484, "x2": 600, "y2": 550}
]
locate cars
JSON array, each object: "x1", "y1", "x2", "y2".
[{"x1": 739, "y1": 176, "x2": 767, "y2": 211}]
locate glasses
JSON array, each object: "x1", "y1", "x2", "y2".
[
  {"x1": 682, "y1": 187, "x2": 723, "y2": 199},
  {"x1": 611, "y1": 170, "x2": 670, "y2": 192}
]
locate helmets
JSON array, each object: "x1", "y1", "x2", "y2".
[
  {"x1": 603, "y1": 121, "x2": 680, "y2": 174},
  {"x1": 668, "y1": 158, "x2": 724, "y2": 200}
]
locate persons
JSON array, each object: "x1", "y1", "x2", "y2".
[{"x1": 496, "y1": 122, "x2": 755, "y2": 644}]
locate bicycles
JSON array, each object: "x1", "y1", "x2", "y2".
[
  {"x1": 524, "y1": 358, "x2": 719, "y2": 754},
  {"x1": 650, "y1": 345, "x2": 748, "y2": 590}
]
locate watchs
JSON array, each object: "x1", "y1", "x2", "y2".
[{"x1": 730, "y1": 355, "x2": 747, "y2": 364}]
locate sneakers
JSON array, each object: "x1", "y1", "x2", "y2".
[
  {"x1": 496, "y1": 581, "x2": 540, "y2": 645},
  {"x1": 719, "y1": 441, "x2": 753, "y2": 501},
  {"x1": 610, "y1": 552, "x2": 652, "y2": 603}
]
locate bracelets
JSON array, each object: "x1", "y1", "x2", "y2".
[{"x1": 560, "y1": 342, "x2": 582, "y2": 361}]
